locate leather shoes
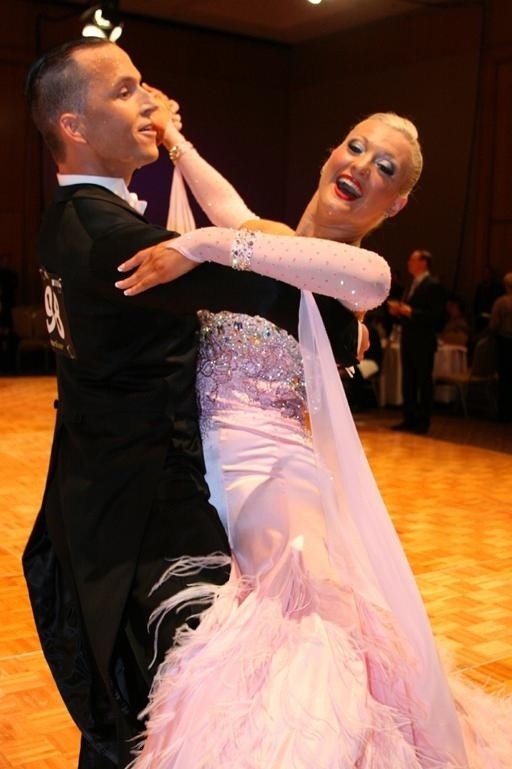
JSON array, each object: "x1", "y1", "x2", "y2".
[{"x1": 390, "y1": 420, "x2": 429, "y2": 434}]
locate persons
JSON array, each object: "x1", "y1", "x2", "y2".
[
  {"x1": 21, "y1": 36, "x2": 376, "y2": 769},
  {"x1": 111, "y1": 77, "x2": 511, "y2": 769},
  {"x1": 351, "y1": 247, "x2": 512, "y2": 437}
]
